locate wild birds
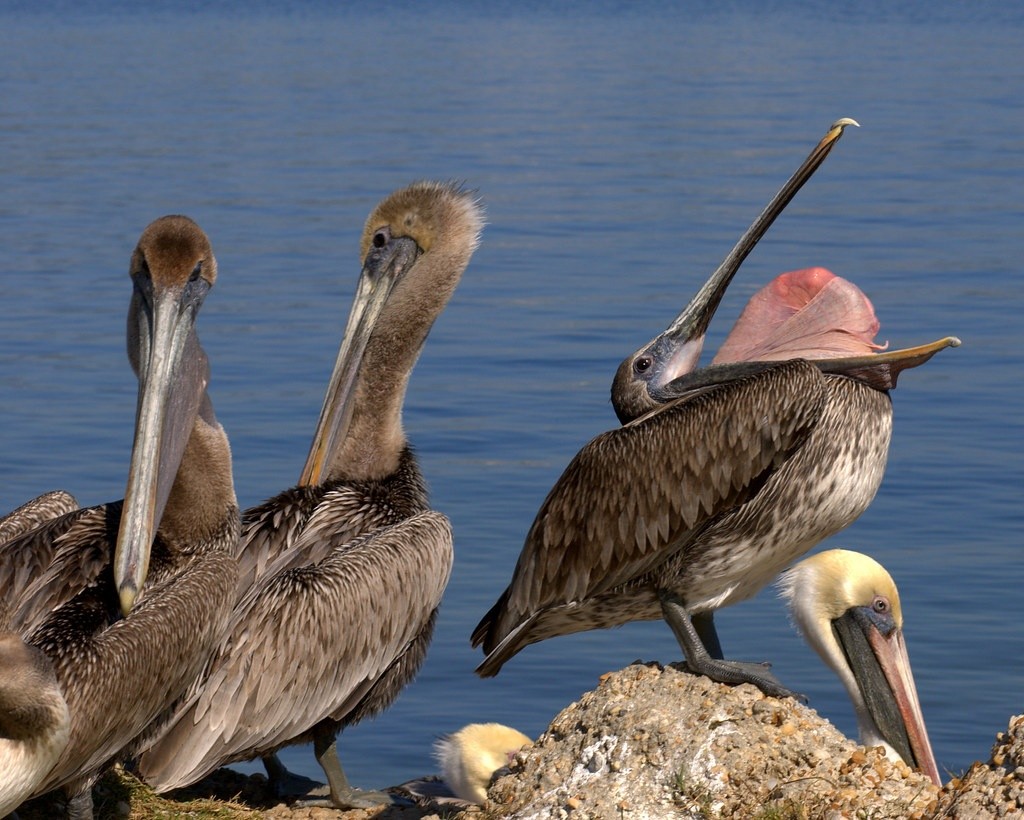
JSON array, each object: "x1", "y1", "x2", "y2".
[
  {"x1": 430, "y1": 117, "x2": 962, "y2": 799},
  {"x1": 1, "y1": 181, "x2": 490, "y2": 820}
]
